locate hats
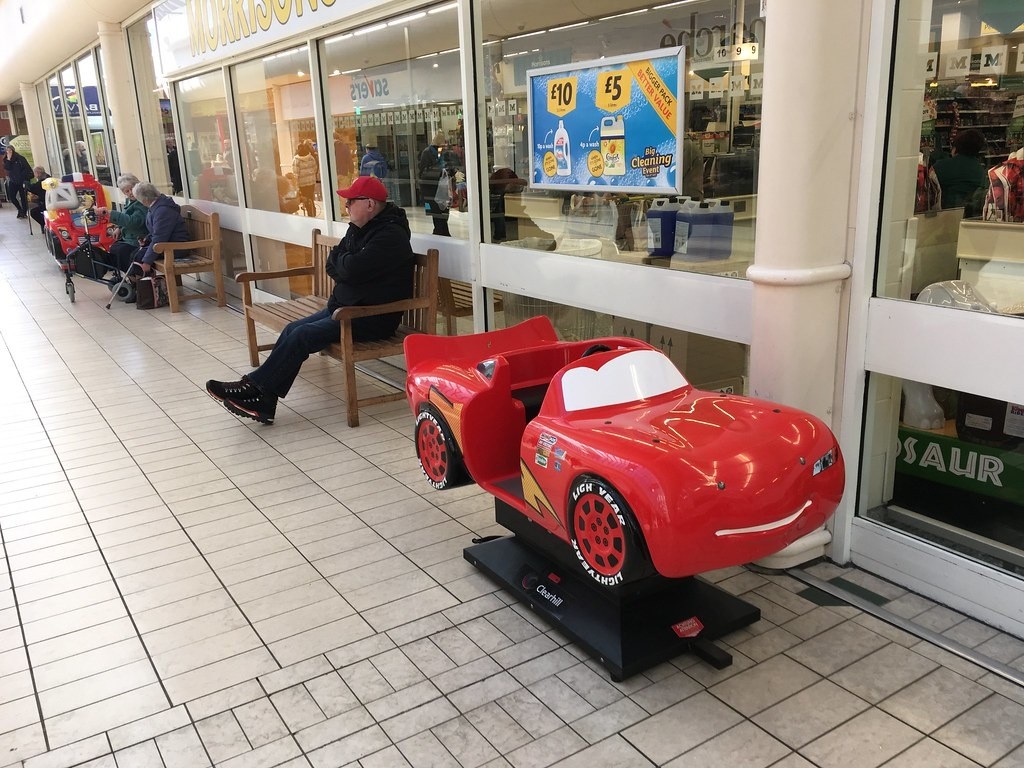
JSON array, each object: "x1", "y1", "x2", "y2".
[
  {"x1": 430, "y1": 128, "x2": 448, "y2": 147},
  {"x1": 6, "y1": 145, "x2": 15, "y2": 151},
  {"x1": 365, "y1": 143, "x2": 377, "y2": 148},
  {"x1": 490, "y1": 168, "x2": 529, "y2": 193},
  {"x1": 336, "y1": 176, "x2": 387, "y2": 202}
]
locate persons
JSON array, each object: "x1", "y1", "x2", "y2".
[
  {"x1": 930, "y1": 129, "x2": 992, "y2": 220},
  {"x1": 188, "y1": 139, "x2": 238, "y2": 201},
  {"x1": 28, "y1": 166, "x2": 51, "y2": 234},
  {"x1": 206, "y1": 177, "x2": 415, "y2": 425},
  {"x1": 2, "y1": 145, "x2": 34, "y2": 218},
  {"x1": 250, "y1": 138, "x2": 319, "y2": 218},
  {"x1": 166, "y1": 138, "x2": 183, "y2": 196},
  {"x1": 416, "y1": 133, "x2": 460, "y2": 238},
  {"x1": 360, "y1": 143, "x2": 388, "y2": 182},
  {"x1": 60, "y1": 140, "x2": 91, "y2": 175},
  {"x1": 93, "y1": 173, "x2": 148, "y2": 274},
  {"x1": 332, "y1": 132, "x2": 356, "y2": 216},
  {"x1": 124, "y1": 182, "x2": 191, "y2": 304}
]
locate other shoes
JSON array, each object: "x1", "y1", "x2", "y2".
[
  {"x1": 17, "y1": 209, "x2": 28, "y2": 219},
  {"x1": 41, "y1": 225, "x2": 45, "y2": 234}
]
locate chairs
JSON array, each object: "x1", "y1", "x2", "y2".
[{"x1": 498, "y1": 337, "x2": 653, "y2": 476}]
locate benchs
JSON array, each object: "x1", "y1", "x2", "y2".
[
  {"x1": 153, "y1": 204, "x2": 225, "y2": 312},
  {"x1": 437, "y1": 272, "x2": 503, "y2": 336},
  {"x1": 235, "y1": 228, "x2": 438, "y2": 426}
]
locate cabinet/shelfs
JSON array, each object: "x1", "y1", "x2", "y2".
[{"x1": 919, "y1": 83, "x2": 1024, "y2": 167}]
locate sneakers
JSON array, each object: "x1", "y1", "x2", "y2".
[{"x1": 206, "y1": 375, "x2": 277, "y2": 425}]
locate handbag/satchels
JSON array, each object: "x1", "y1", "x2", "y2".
[
  {"x1": 434, "y1": 169, "x2": 454, "y2": 213},
  {"x1": 137, "y1": 267, "x2": 170, "y2": 310}
]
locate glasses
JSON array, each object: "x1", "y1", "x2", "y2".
[
  {"x1": 509, "y1": 186, "x2": 524, "y2": 193},
  {"x1": 347, "y1": 198, "x2": 370, "y2": 205}
]
